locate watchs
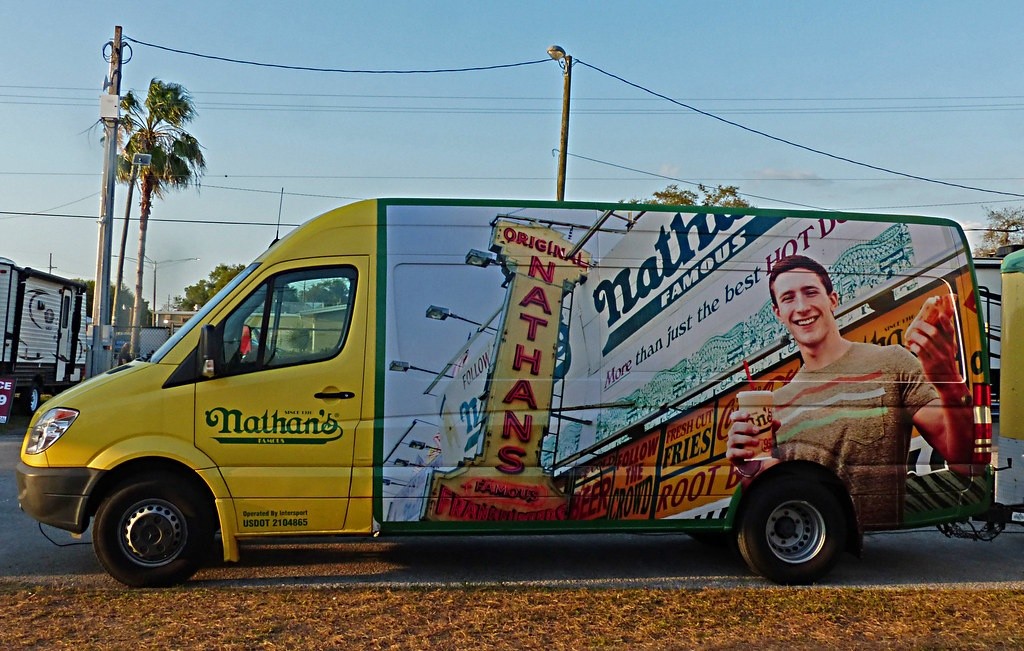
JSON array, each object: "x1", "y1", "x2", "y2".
[{"x1": 943, "y1": 389, "x2": 973, "y2": 409}]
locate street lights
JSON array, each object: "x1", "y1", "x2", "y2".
[
  {"x1": 548, "y1": 44, "x2": 572, "y2": 202},
  {"x1": 112, "y1": 253, "x2": 200, "y2": 327}
]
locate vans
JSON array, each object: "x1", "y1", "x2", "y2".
[{"x1": 17, "y1": 187, "x2": 994, "y2": 584}]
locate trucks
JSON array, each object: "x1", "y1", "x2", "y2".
[{"x1": 0, "y1": 256, "x2": 88, "y2": 415}]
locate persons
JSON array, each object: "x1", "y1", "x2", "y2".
[
  {"x1": 725, "y1": 256, "x2": 977, "y2": 527},
  {"x1": 118, "y1": 343, "x2": 132, "y2": 365},
  {"x1": 147, "y1": 350, "x2": 155, "y2": 363}
]
